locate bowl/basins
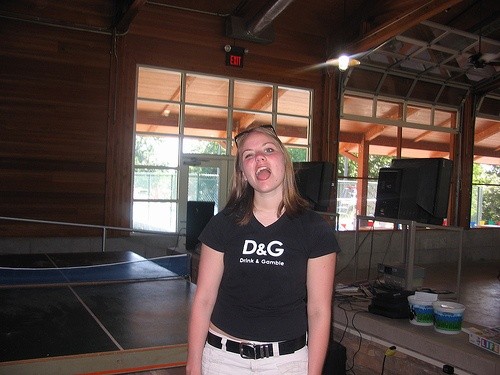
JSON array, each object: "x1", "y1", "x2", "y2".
[
  {"x1": 433, "y1": 300, "x2": 465, "y2": 335},
  {"x1": 408, "y1": 292, "x2": 440, "y2": 326}
]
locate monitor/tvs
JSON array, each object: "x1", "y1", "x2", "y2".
[
  {"x1": 375, "y1": 158, "x2": 453, "y2": 226},
  {"x1": 297, "y1": 161, "x2": 334, "y2": 211}
]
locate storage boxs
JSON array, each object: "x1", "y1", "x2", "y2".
[{"x1": 470, "y1": 326, "x2": 500, "y2": 355}]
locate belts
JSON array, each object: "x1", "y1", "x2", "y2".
[{"x1": 207, "y1": 330, "x2": 307, "y2": 360}]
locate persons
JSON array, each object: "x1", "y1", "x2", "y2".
[{"x1": 184, "y1": 124, "x2": 342, "y2": 375}]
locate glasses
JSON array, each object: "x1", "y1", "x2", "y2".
[{"x1": 234, "y1": 124, "x2": 278, "y2": 149}]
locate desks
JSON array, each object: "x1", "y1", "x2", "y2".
[
  {"x1": 356, "y1": 215, "x2": 464, "y2": 300},
  {"x1": 0, "y1": 251, "x2": 198, "y2": 375}
]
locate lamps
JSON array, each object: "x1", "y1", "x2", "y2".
[{"x1": 225, "y1": 45, "x2": 249, "y2": 65}]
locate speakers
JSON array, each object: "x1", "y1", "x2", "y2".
[{"x1": 186, "y1": 201, "x2": 216, "y2": 250}]
